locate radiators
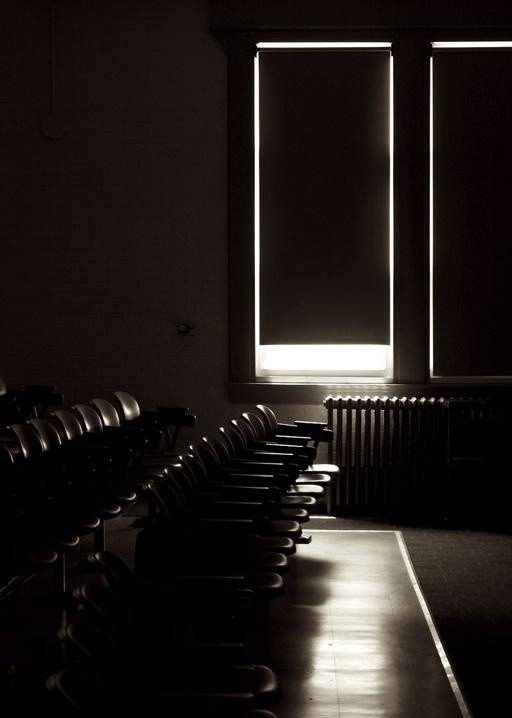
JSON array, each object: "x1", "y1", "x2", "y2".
[{"x1": 323, "y1": 396, "x2": 487, "y2": 516}]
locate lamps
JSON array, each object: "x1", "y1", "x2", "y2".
[{"x1": 254, "y1": 37, "x2": 512, "y2": 379}]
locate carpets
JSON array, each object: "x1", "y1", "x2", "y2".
[{"x1": 401, "y1": 519, "x2": 511, "y2": 718}]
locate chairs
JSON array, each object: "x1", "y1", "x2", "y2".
[{"x1": 0, "y1": 385, "x2": 340, "y2": 718}]
